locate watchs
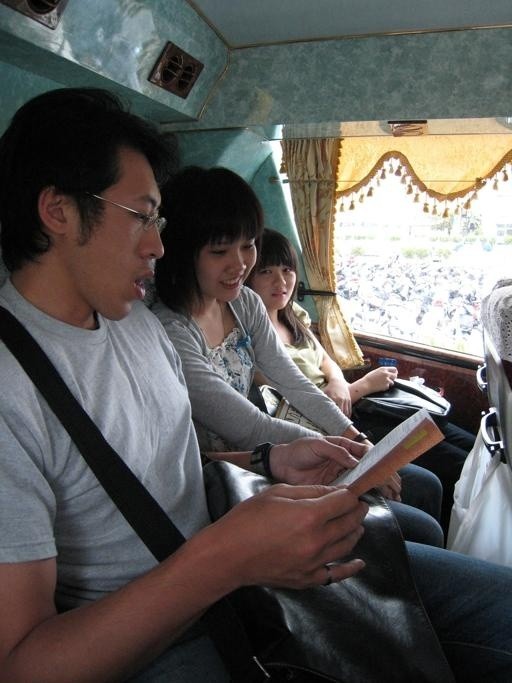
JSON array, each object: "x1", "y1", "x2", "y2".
[
  {"x1": 251, "y1": 442, "x2": 278, "y2": 482},
  {"x1": 354, "y1": 431, "x2": 369, "y2": 443},
  {"x1": 141, "y1": 165, "x2": 444, "y2": 548}
]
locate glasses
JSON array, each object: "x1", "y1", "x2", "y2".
[{"x1": 94, "y1": 193, "x2": 166, "y2": 230}]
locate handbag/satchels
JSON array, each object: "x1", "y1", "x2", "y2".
[
  {"x1": 353, "y1": 378, "x2": 451, "y2": 425},
  {"x1": 0, "y1": 309, "x2": 456, "y2": 683}
]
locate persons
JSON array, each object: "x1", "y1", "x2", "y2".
[
  {"x1": 247, "y1": 229, "x2": 478, "y2": 488},
  {"x1": 0, "y1": 88, "x2": 512, "y2": 683}
]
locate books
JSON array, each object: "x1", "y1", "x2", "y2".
[{"x1": 327, "y1": 407, "x2": 446, "y2": 496}]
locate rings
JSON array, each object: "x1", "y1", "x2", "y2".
[{"x1": 322, "y1": 565, "x2": 333, "y2": 586}]
